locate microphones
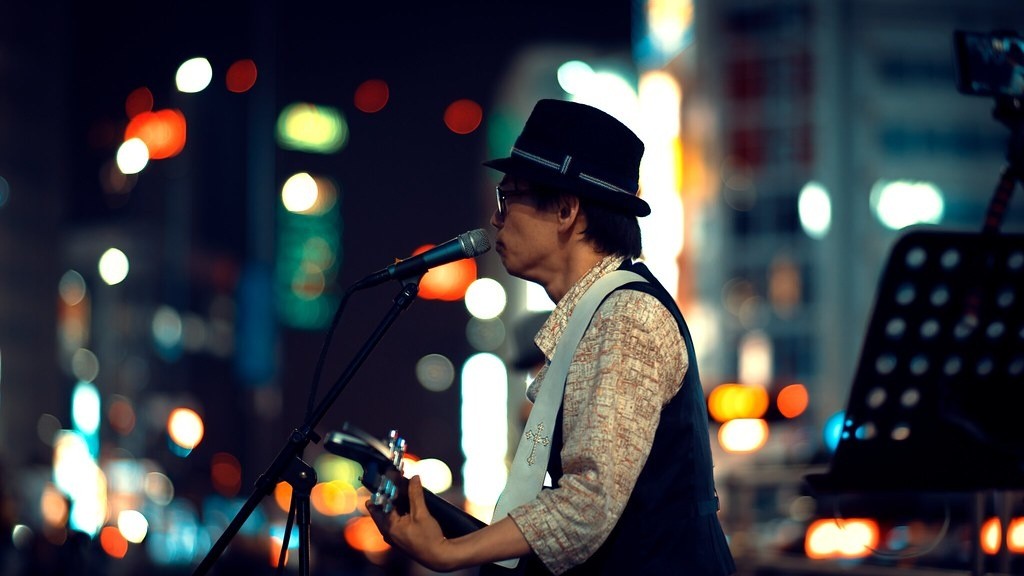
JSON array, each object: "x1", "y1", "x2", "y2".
[{"x1": 356, "y1": 229, "x2": 492, "y2": 290}]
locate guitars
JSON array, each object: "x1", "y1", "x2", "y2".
[{"x1": 324, "y1": 422, "x2": 558, "y2": 576}]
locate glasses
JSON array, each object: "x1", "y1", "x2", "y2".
[{"x1": 495, "y1": 186, "x2": 574, "y2": 215}]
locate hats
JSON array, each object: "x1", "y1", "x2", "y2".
[{"x1": 482, "y1": 98, "x2": 651, "y2": 217}]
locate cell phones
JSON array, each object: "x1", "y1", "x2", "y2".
[{"x1": 952, "y1": 28, "x2": 1024, "y2": 98}]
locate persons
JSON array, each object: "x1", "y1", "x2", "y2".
[{"x1": 364, "y1": 100, "x2": 738, "y2": 576}]
